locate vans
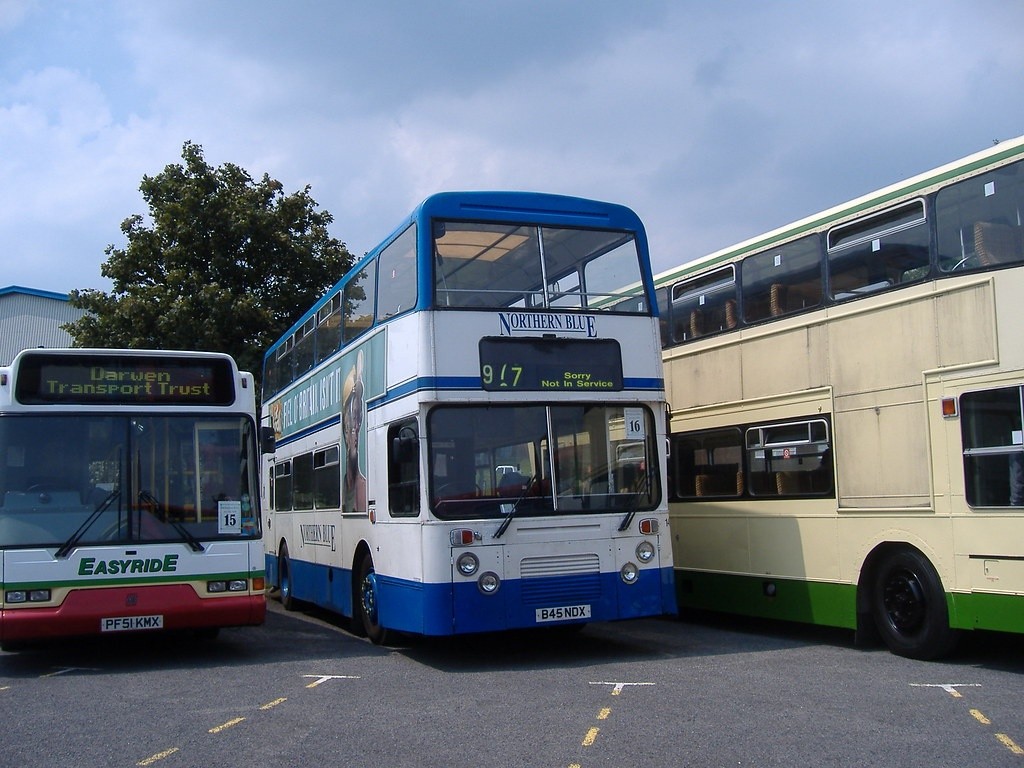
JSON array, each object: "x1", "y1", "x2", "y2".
[{"x1": 495, "y1": 466, "x2": 515, "y2": 475}]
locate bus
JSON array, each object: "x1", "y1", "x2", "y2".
[
  {"x1": 259, "y1": 191, "x2": 676, "y2": 645},
  {"x1": 526, "y1": 134, "x2": 1024, "y2": 660},
  {"x1": 0, "y1": 348, "x2": 275, "y2": 655}
]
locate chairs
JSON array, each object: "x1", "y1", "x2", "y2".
[
  {"x1": 777, "y1": 471, "x2": 802, "y2": 495},
  {"x1": 974, "y1": 220, "x2": 1020, "y2": 266},
  {"x1": 690, "y1": 310, "x2": 714, "y2": 337},
  {"x1": 737, "y1": 463, "x2": 764, "y2": 495},
  {"x1": 770, "y1": 284, "x2": 802, "y2": 315},
  {"x1": 695, "y1": 474, "x2": 718, "y2": 497},
  {"x1": 725, "y1": 298, "x2": 754, "y2": 330},
  {"x1": 1009, "y1": 453, "x2": 1024, "y2": 506},
  {"x1": 659, "y1": 320, "x2": 678, "y2": 348}
]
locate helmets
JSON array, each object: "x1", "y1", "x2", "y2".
[{"x1": 342, "y1": 367, "x2": 364, "y2": 420}]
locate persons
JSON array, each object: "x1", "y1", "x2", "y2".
[{"x1": 342, "y1": 365, "x2": 366, "y2": 513}]
